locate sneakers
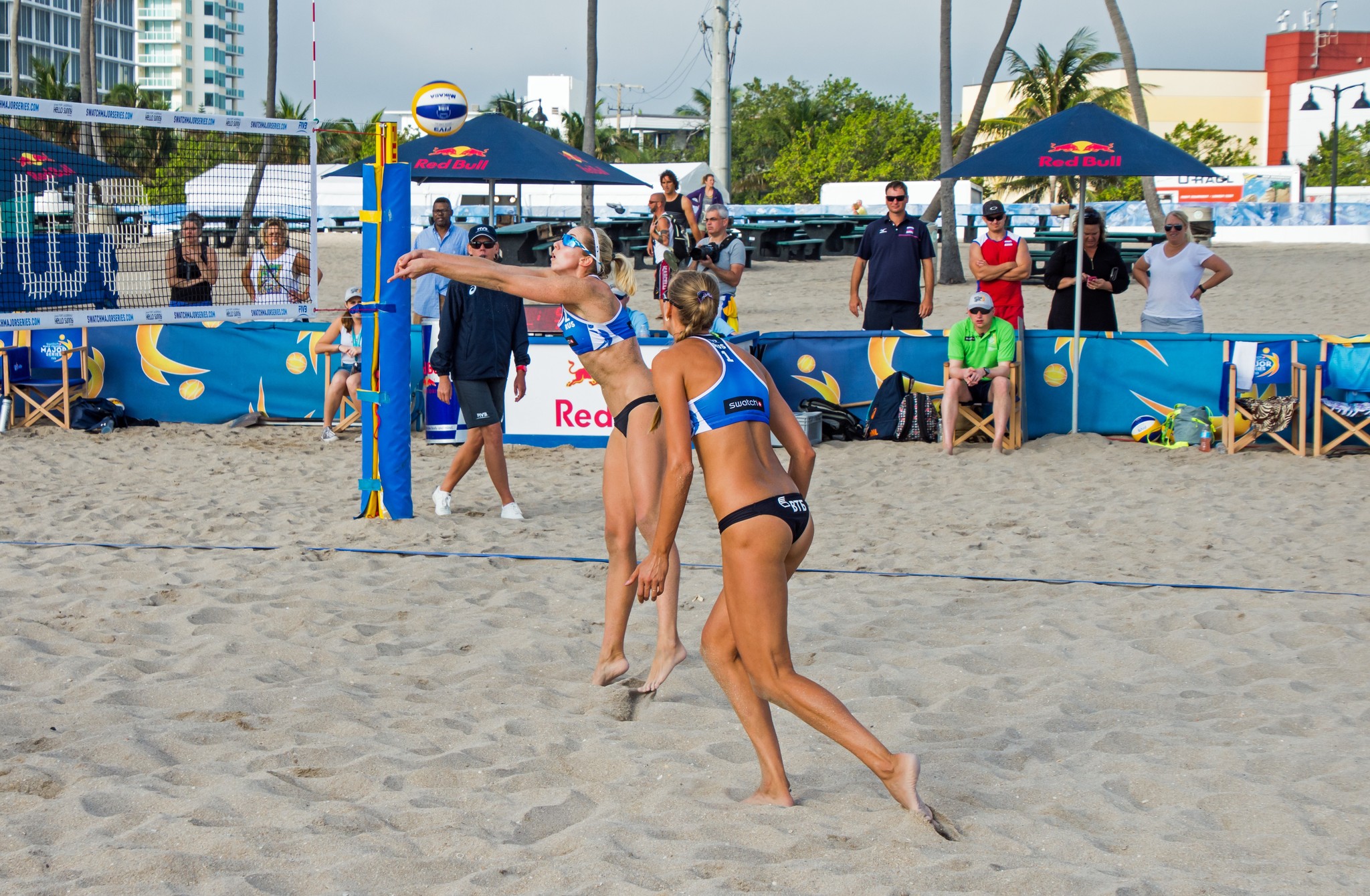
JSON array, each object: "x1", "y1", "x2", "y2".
[
  {"x1": 321, "y1": 425, "x2": 340, "y2": 442},
  {"x1": 355, "y1": 434, "x2": 362, "y2": 443},
  {"x1": 432, "y1": 485, "x2": 451, "y2": 516},
  {"x1": 500, "y1": 502, "x2": 525, "y2": 519}
]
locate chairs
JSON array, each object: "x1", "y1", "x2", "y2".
[
  {"x1": 1313, "y1": 340, "x2": 1370, "y2": 457},
  {"x1": 1219, "y1": 339, "x2": 1306, "y2": 457},
  {"x1": 943, "y1": 341, "x2": 1022, "y2": 450},
  {"x1": 324, "y1": 350, "x2": 361, "y2": 432},
  {"x1": 0, "y1": 326, "x2": 88, "y2": 429}
]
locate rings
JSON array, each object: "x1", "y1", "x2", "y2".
[{"x1": 1093, "y1": 286, "x2": 1097, "y2": 290}]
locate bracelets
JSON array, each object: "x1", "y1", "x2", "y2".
[
  {"x1": 1199, "y1": 284, "x2": 1206, "y2": 293},
  {"x1": 650, "y1": 231, "x2": 653, "y2": 236},
  {"x1": 338, "y1": 344, "x2": 342, "y2": 352}
]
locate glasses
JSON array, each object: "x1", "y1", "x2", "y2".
[
  {"x1": 886, "y1": 195, "x2": 907, "y2": 202},
  {"x1": 1164, "y1": 224, "x2": 1183, "y2": 232},
  {"x1": 703, "y1": 217, "x2": 725, "y2": 223},
  {"x1": 1083, "y1": 212, "x2": 1100, "y2": 218},
  {"x1": 649, "y1": 200, "x2": 663, "y2": 204},
  {"x1": 561, "y1": 234, "x2": 603, "y2": 267},
  {"x1": 663, "y1": 290, "x2": 682, "y2": 309},
  {"x1": 970, "y1": 307, "x2": 993, "y2": 315},
  {"x1": 986, "y1": 213, "x2": 1004, "y2": 222},
  {"x1": 432, "y1": 209, "x2": 451, "y2": 214},
  {"x1": 470, "y1": 241, "x2": 496, "y2": 249}
]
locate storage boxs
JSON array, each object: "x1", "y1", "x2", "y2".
[{"x1": 769, "y1": 412, "x2": 822, "y2": 447}]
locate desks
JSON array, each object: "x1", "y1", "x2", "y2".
[
  {"x1": 963, "y1": 214, "x2": 1055, "y2": 231},
  {"x1": 1023, "y1": 231, "x2": 1168, "y2": 252},
  {"x1": 422, "y1": 211, "x2": 654, "y2": 266},
  {"x1": 283, "y1": 218, "x2": 323, "y2": 227},
  {"x1": 116, "y1": 211, "x2": 150, "y2": 234},
  {"x1": 176, "y1": 215, "x2": 287, "y2": 248},
  {"x1": 34, "y1": 211, "x2": 77, "y2": 227},
  {"x1": 727, "y1": 213, "x2": 884, "y2": 261},
  {"x1": 330, "y1": 216, "x2": 359, "y2": 226}
]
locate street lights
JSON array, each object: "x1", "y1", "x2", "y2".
[
  {"x1": 491, "y1": 96, "x2": 550, "y2": 223},
  {"x1": 1299, "y1": 82, "x2": 1370, "y2": 226}
]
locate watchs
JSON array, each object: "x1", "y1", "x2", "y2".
[
  {"x1": 983, "y1": 367, "x2": 990, "y2": 377},
  {"x1": 516, "y1": 365, "x2": 527, "y2": 372}
]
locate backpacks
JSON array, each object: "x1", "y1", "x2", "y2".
[
  {"x1": 653, "y1": 214, "x2": 691, "y2": 263},
  {"x1": 895, "y1": 393, "x2": 938, "y2": 443}
]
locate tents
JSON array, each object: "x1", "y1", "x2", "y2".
[{"x1": 183, "y1": 161, "x2": 732, "y2": 218}]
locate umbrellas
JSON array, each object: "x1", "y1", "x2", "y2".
[
  {"x1": 320, "y1": 112, "x2": 654, "y2": 228},
  {"x1": 0, "y1": 123, "x2": 142, "y2": 206},
  {"x1": 933, "y1": 103, "x2": 1222, "y2": 438}
]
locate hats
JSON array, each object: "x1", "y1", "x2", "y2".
[
  {"x1": 983, "y1": 199, "x2": 1006, "y2": 216},
  {"x1": 344, "y1": 286, "x2": 362, "y2": 301},
  {"x1": 468, "y1": 224, "x2": 497, "y2": 243},
  {"x1": 968, "y1": 291, "x2": 994, "y2": 310}
]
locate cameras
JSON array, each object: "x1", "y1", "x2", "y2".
[{"x1": 690, "y1": 242, "x2": 720, "y2": 261}]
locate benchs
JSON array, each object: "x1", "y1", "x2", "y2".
[
  {"x1": 619, "y1": 227, "x2": 868, "y2": 270},
  {"x1": 955, "y1": 225, "x2": 1061, "y2": 243},
  {"x1": 532, "y1": 239, "x2": 561, "y2": 266},
  {"x1": 1018, "y1": 248, "x2": 1151, "y2": 285},
  {"x1": 167, "y1": 225, "x2": 362, "y2": 249},
  {"x1": 33, "y1": 221, "x2": 156, "y2": 244}
]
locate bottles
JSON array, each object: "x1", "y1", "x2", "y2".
[
  {"x1": 1216, "y1": 442, "x2": 1226, "y2": 454},
  {"x1": 937, "y1": 418, "x2": 943, "y2": 443},
  {"x1": 1200, "y1": 424, "x2": 1211, "y2": 452},
  {"x1": 639, "y1": 325, "x2": 648, "y2": 338},
  {"x1": 102, "y1": 420, "x2": 114, "y2": 434}
]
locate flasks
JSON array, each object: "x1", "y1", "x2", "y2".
[{"x1": 0, "y1": 394, "x2": 13, "y2": 432}]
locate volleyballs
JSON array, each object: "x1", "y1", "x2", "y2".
[
  {"x1": 1130, "y1": 415, "x2": 1161, "y2": 444},
  {"x1": 106, "y1": 397, "x2": 125, "y2": 411},
  {"x1": 411, "y1": 79, "x2": 469, "y2": 138}
]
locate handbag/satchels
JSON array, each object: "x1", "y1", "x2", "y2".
[
  {"x1": 69, "y1": 396, "x2": 128, "y2": 433},
  {"x1": 1147, "y1": 403, "x2": 1216, "y2": 449},
  {"x1": 863, "y1": 371, "x2": 915, "y2": 440},
  {"x1": 797, "y1": 397, "x2": 864, "y2": 442}
]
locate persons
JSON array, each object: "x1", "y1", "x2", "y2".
[
  {"x1": 850, "y1": 181, "x2": 937, "y2": 331},
  {"x1": 315, "y1": 285, "x2": 363, "y2": 442},
  {"x1": 240, "y1": 216, "x2": 323, "y2": 324},
  {"x1": 165, "y1": 212, "x2": 218, "y2": 307},
  {"x1": 432, "y1": 227, "x2": 531, "y2": 520},
  {"x1": 608, "y1": 280, "x2": 650, "y2": 338},
  {"x1": 969, "y1": 199, "x2": 1033, "y2": 331},
  {"x1": 385, "y1": 223, "x2": 688, "y2": 694},
  {"x1": 647, "y1": 169, "x2": 746, "y2": 338},
  {"x1": 413, "y1": 197, "x2": 470, "y2": 324},
  {"x1": 1131, "y1": 209, "x2": 1235, "y2": 335},
  {"x1": 851, "y1": 200, "x2": 867, "y2": 215},
  {"x1": 1043, "y1": 207, "x2": 1131, "y2": 332},
  {"x1": 940, "y1": 291, "x2": 1016, "y2": 456},
  {"x1": 622, "y1": 270, "x2": 934, "y2": 826}
]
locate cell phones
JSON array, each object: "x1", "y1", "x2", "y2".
[{"x1": 1088, "y1": 277, "x2": 1097, "y2": 281}]
